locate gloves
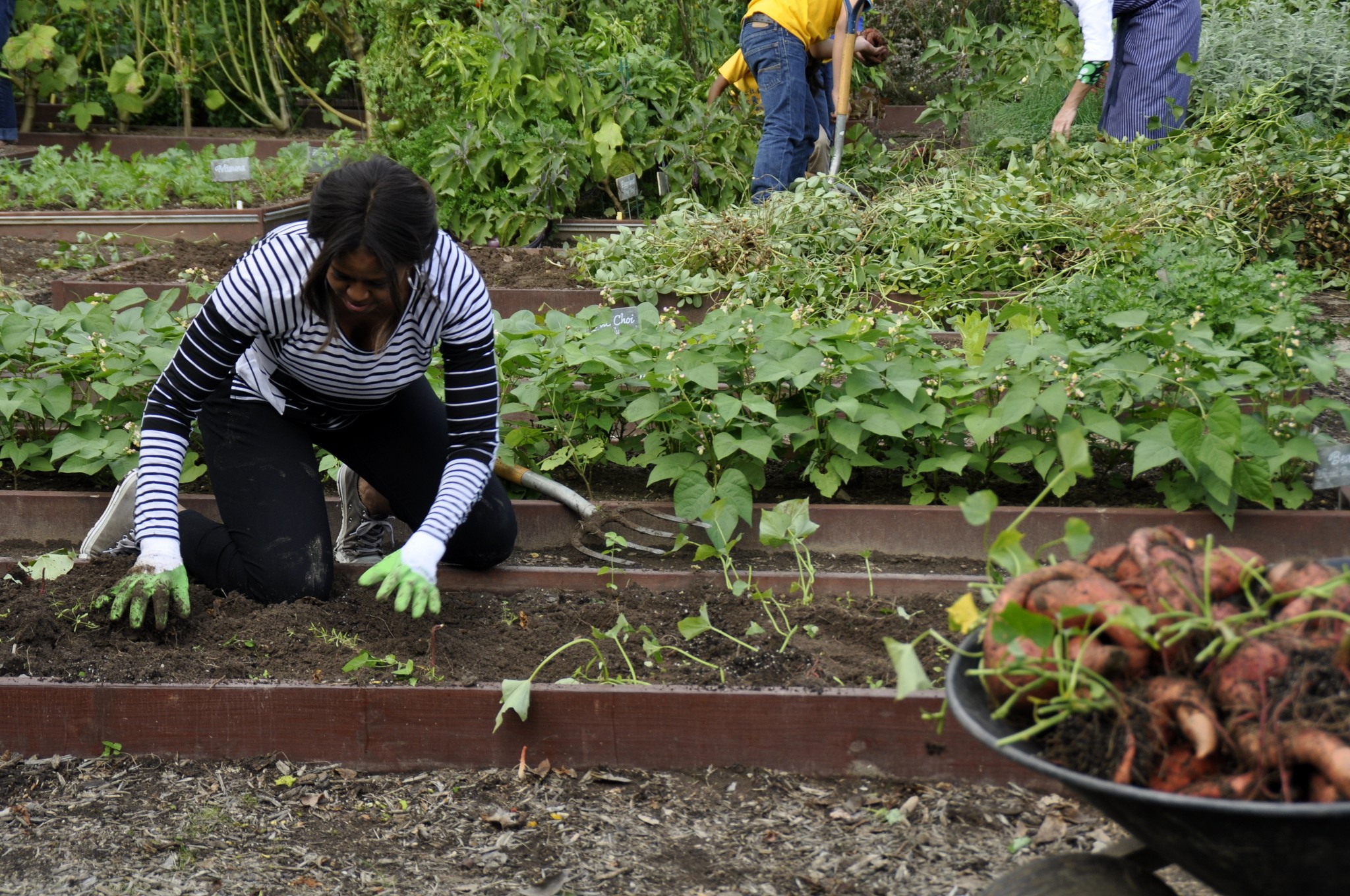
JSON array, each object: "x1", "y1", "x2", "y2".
[
  {"x1": 95, "y1": 536, "x2": 191, "y2": 630},
  {"x1": 358, "y1": 531, "x2": 446, "y2": 622}
]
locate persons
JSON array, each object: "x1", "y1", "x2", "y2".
[
  {"x1": 741, "y1": 0, "x2": 886, "y2": 205},
  {"x1": 707, "y1": 49, "x2": 764, "y2": 114},
  {"x1": 1051, "y1": 0, "x2": 1202, "y2": 139},
  {"x1": 92, "y1": 155, "x2": 520, "y2": 619}
]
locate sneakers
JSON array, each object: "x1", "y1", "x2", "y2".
[
  {"x1": 333, "y1": 462, "x2": 392, "y2": 567},
  {"x1": 80, "y1": 467, "x2": 139, "y2": 563}
]
